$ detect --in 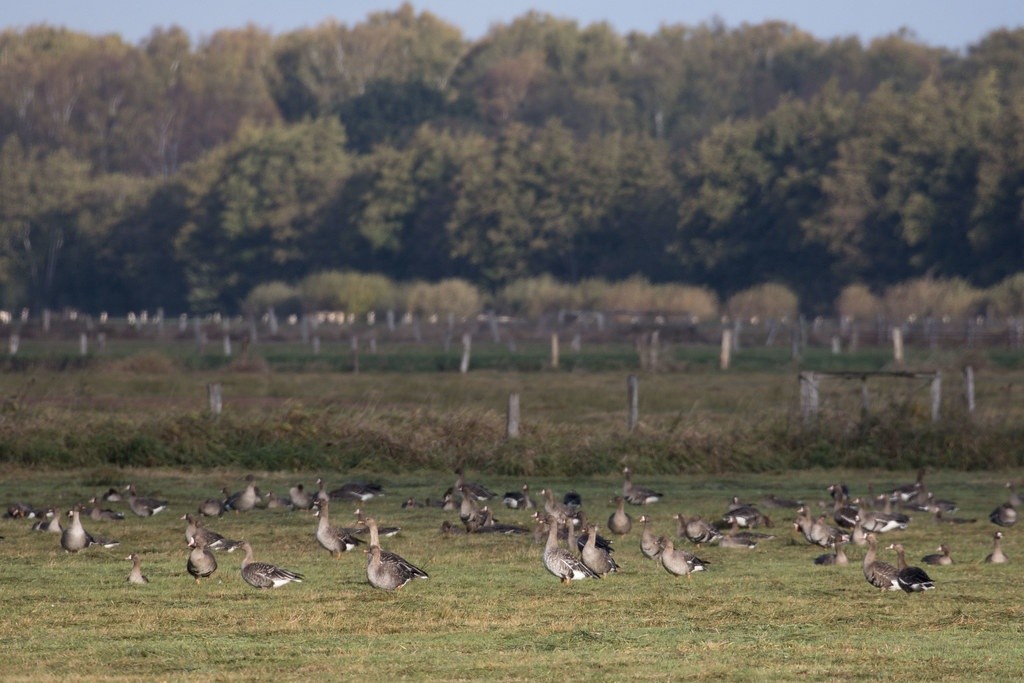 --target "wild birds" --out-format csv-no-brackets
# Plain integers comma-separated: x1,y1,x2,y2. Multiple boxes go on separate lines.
0,462,1020,600
